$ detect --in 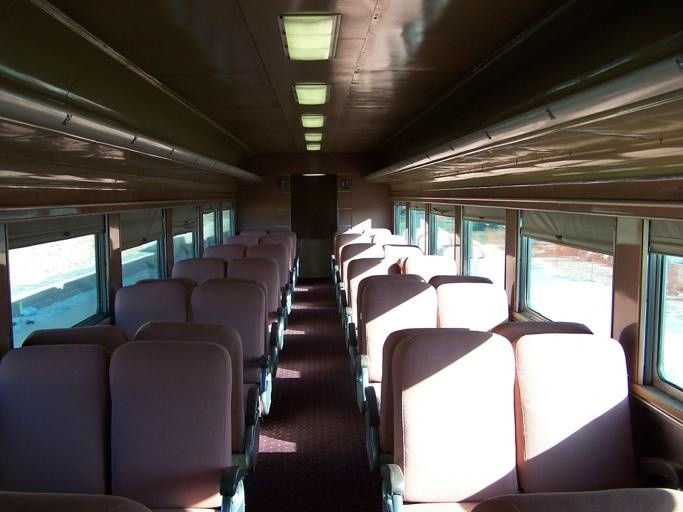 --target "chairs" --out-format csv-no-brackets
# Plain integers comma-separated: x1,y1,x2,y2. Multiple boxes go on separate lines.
330,223,680,512
2,227,300,511
